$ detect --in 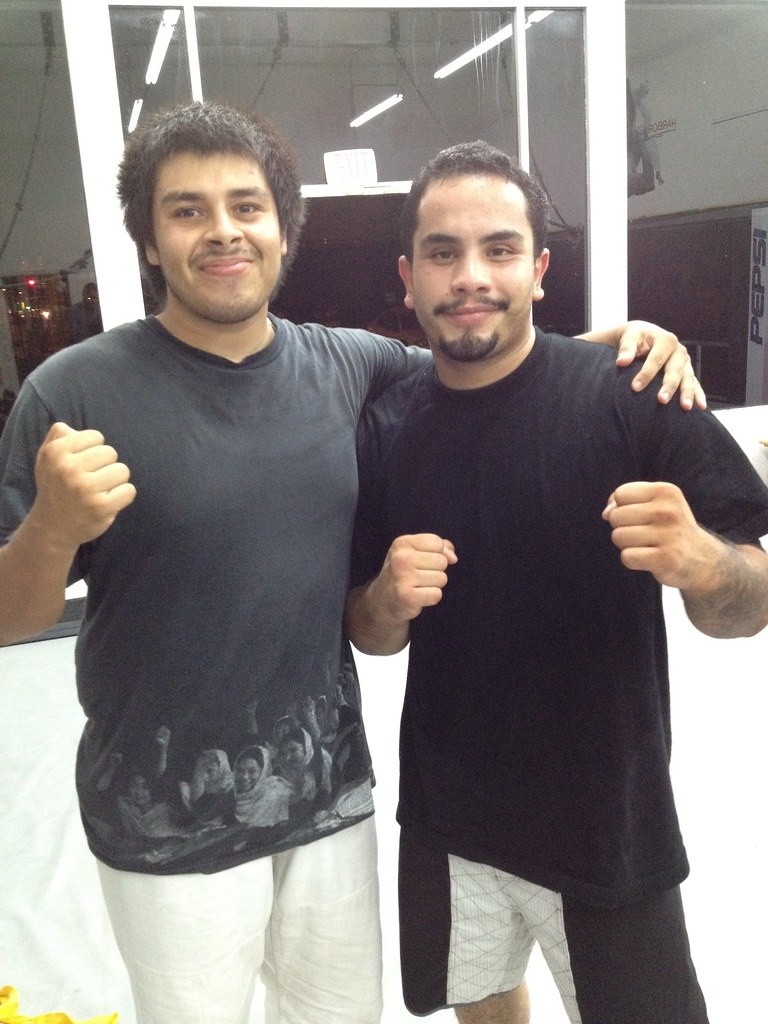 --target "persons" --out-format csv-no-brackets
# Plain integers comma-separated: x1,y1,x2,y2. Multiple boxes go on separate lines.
1,102,707,1023
61,280,103,349
340,135,768,1023
